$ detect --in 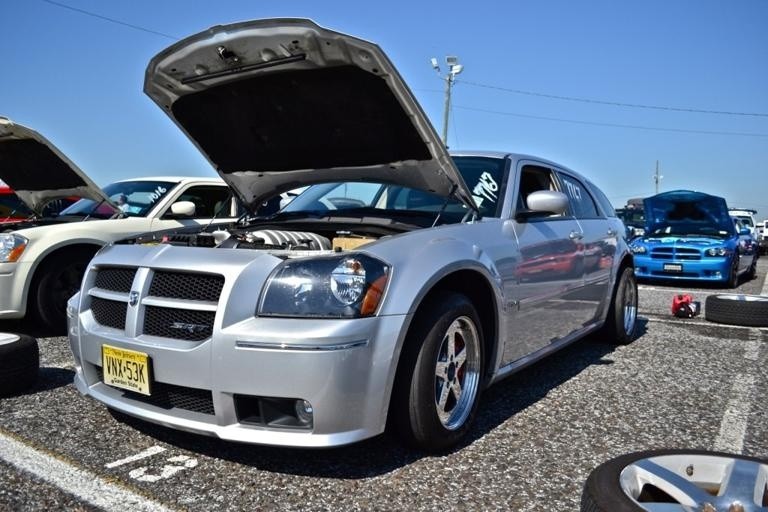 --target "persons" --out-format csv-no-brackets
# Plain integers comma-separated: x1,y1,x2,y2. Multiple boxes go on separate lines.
116,195,132,214
